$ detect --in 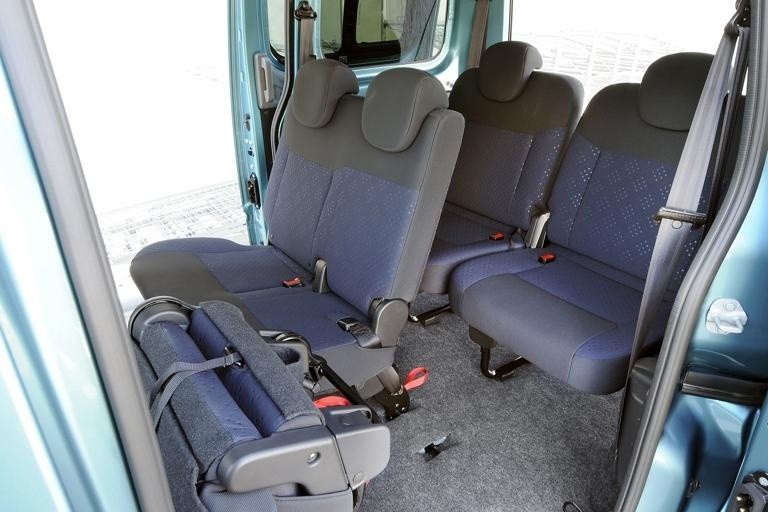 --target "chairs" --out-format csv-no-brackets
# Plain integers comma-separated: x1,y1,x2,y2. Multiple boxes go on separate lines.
129,59,466,512
406,41,713,398
